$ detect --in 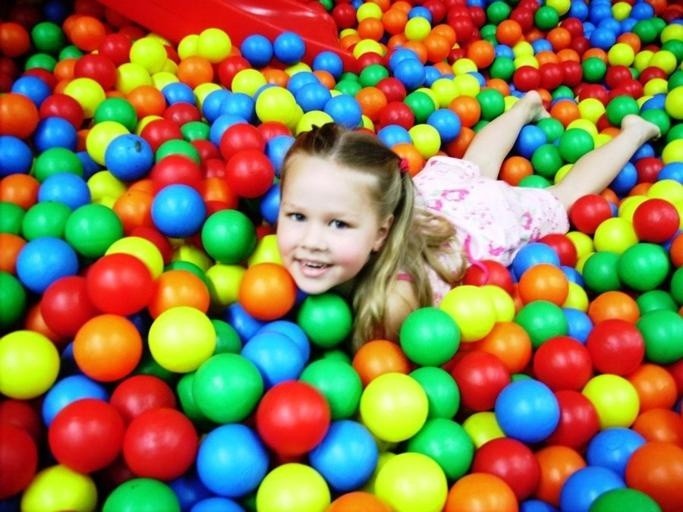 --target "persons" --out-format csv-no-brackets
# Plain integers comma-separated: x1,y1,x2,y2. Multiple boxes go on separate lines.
277,90,661,351
